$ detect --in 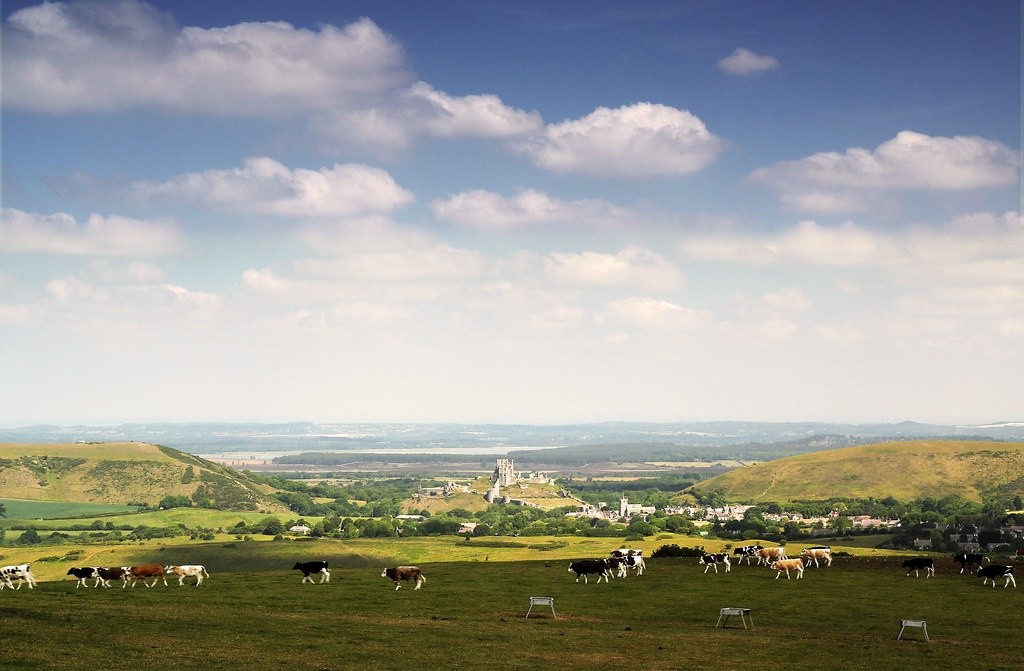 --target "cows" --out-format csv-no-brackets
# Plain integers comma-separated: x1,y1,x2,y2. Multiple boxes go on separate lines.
567,549,647,584
292,560,331,585
0,564,38,590
66,562,210,589
954,553,990,575
733,545,832,580
699,553,732,574
977,565,1017,588
381,566,427,591
901,557,936,579
